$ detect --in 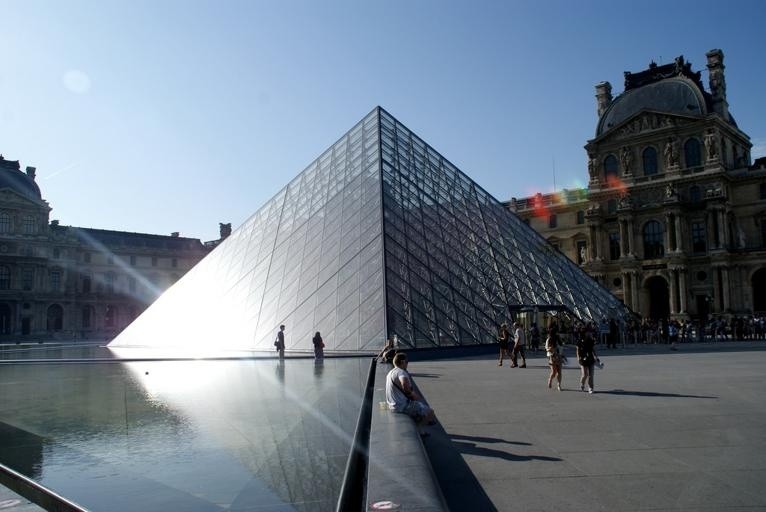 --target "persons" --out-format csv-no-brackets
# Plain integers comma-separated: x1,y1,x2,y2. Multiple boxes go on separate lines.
278,324,287,357
497,322,518,367
274,358,286,383
546,327,565,392
373,339,393,363
496,313,766,351
383,345,399,361
510,321,527,368
312,330,325,358
313,355,324,379
576,327,601,394
384,352,438,436
